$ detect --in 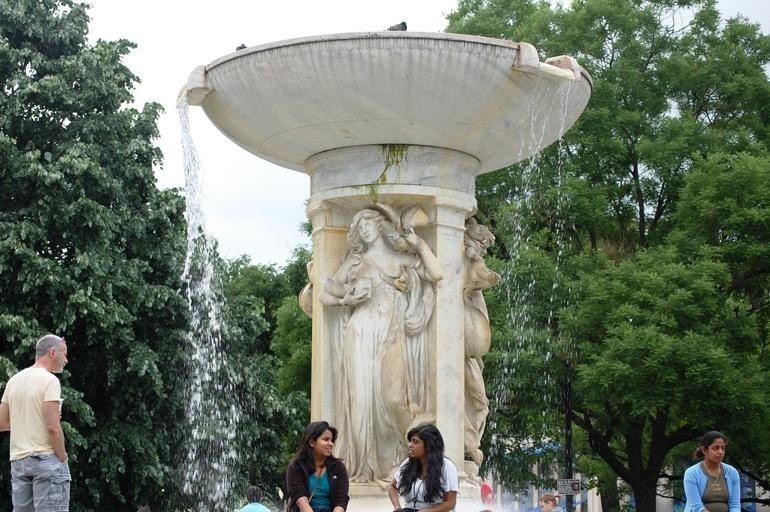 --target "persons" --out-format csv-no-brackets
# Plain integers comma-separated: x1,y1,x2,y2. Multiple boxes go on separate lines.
386,422,462,511
284,421,350,512
240,484,272,511
322,209,443,489
682,431,742,511
539,493,567,511
0,333,74,512
466,220,503,472
